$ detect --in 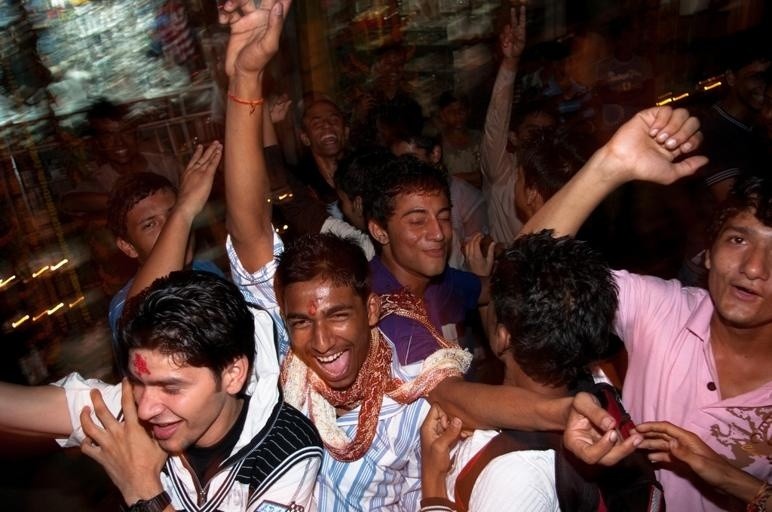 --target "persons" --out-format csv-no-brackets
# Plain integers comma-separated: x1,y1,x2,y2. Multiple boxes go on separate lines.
1,0,771,512
